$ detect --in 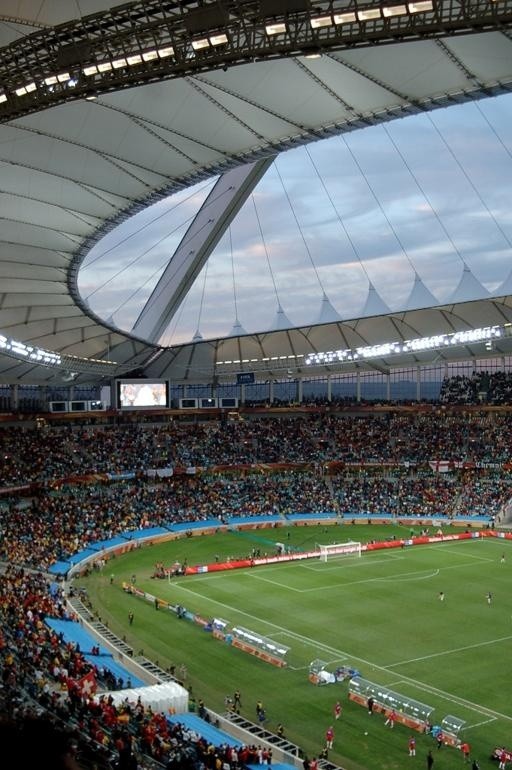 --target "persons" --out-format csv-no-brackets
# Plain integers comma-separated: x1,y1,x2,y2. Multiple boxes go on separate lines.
1,562,512,769
1,370,512,579
